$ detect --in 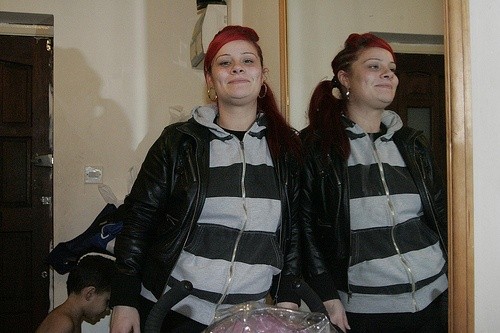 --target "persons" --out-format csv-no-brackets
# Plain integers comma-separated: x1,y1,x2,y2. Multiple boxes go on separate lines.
110,26,302,333
300,33,448,333
36,255,114,333
